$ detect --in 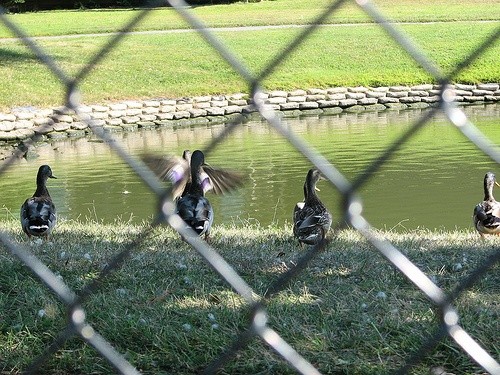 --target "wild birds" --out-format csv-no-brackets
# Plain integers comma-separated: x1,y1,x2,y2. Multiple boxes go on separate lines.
20,165,58,243
142,149,250,203
292,170,333,248
473,171,500,239
175,150,215,244
